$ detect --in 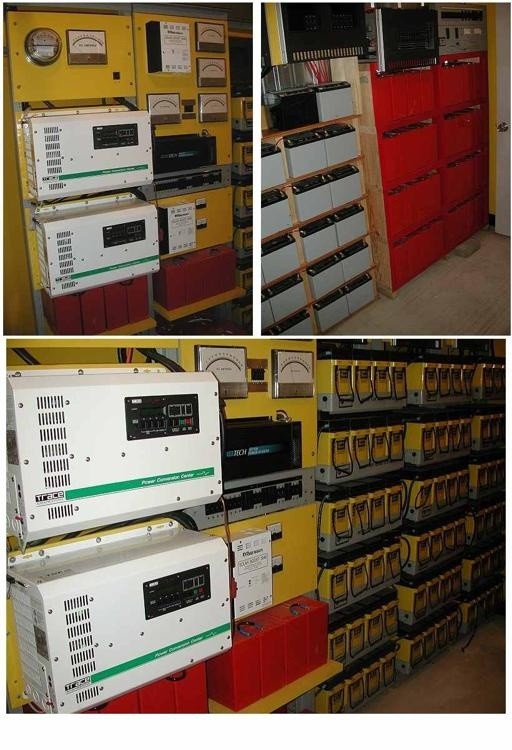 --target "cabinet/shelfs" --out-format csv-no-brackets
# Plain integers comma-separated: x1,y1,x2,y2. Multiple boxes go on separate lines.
262,50,488,335
